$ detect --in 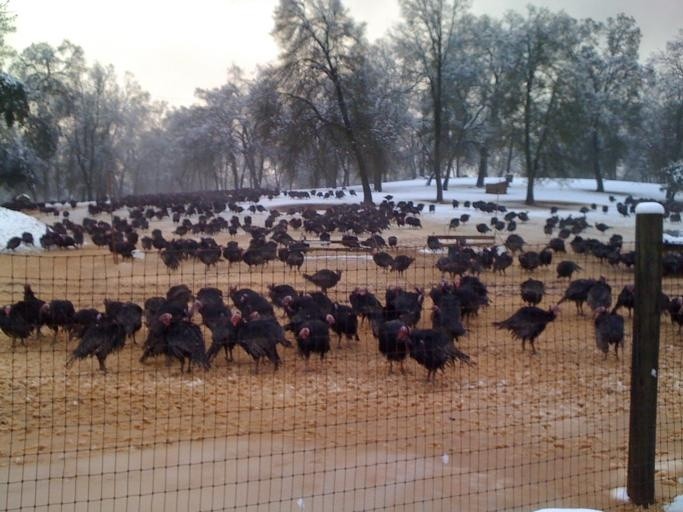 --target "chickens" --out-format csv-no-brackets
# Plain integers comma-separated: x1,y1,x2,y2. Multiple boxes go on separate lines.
0,188,683,390
